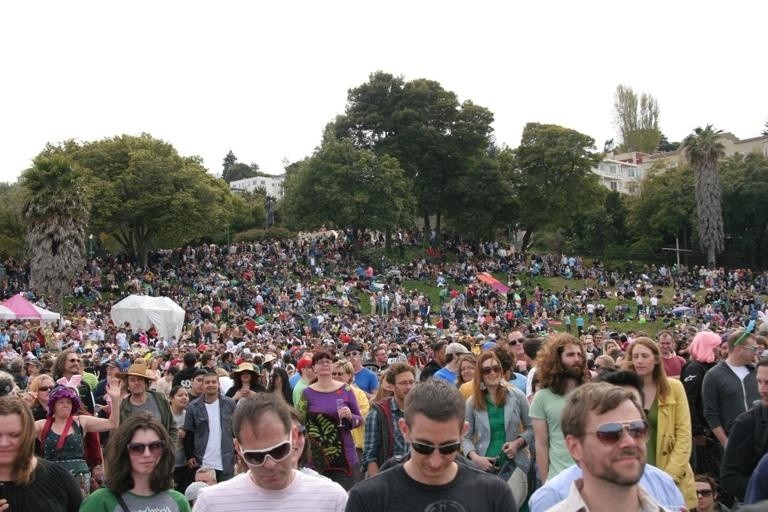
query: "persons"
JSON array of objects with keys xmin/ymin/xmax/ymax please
[{"xmin": 0, "ymin": 223, "xmax": 768, "ymax": 511}]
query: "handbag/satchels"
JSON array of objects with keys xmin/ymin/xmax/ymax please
[{"xmin": 488, "ymin": 451, "xmax": 518, "ymax": 482}]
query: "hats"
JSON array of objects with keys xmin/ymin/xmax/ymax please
[
  {"xmin": 296, "ymin": 342, "xmax": 474, "ymax": 371},
  {"xmin": 104, "ymin": 354, "xmax": 278, "ymax": 381},
  {"xmin": 47, "ymin": 385, "xmax": 80, "ymax": 415}
]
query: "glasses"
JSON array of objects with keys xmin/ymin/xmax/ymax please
[
  {"xmin": 695, "ymin": 489, "xmax": 714, "ymax": 498},
  {"xmin": 236, "ymin": 422, "xmax": 293, "ymax": 467},
  {"xmin": 332, "ymin": 372, "xmax": 343, "ymax": 376},
  {"xmin": 126, "ymin": 441, "xmax": 162, "ymax": 457},
  {"xmin": 742, "ymin": 344, "xmax": 759, "ymax": 353},
  {"xmin": 406, "ymin": 423, "xmax": 464, "ymax": 455},
  {"xmin": 507, "ymin": 337, "xmax": 525, "ymax": 346},
  {"xmin": 481, "ymin": 363, "xmax": 502, "ymax": 374},
  {"xmin": 69, "ymin": 358, "xmax": 80, "ymax": 363},
  {"xmin": 293, "ymin": 424, "xmax": 308, "ymax": 439},
  {"xmin": 582, "ymin": 420, "xmax": 652, "ymax": 447},
  {"xmin": 39, "ymin": 385, "xmax": 53, "ymax": 391}
]
